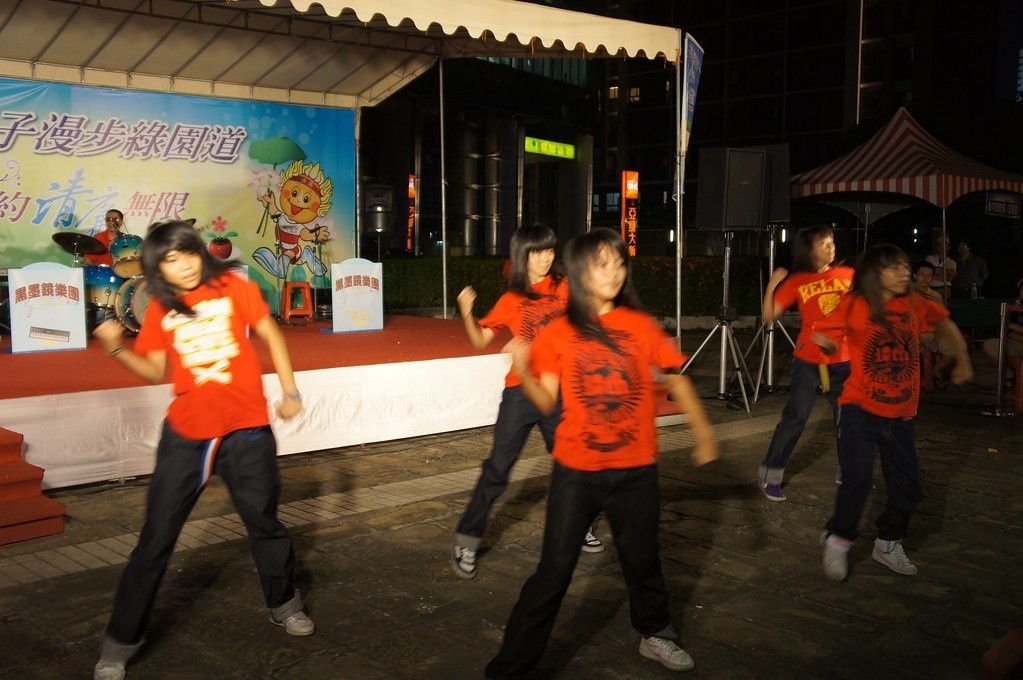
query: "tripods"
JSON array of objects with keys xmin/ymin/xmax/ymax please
[{"xmin": 677, "ymin": 226, "xmax": 795, "ymax": 418}]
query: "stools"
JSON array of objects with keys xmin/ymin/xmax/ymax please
[{"xmin": 283, "ymin": 282, "xmax": 313, "ymax": 324}]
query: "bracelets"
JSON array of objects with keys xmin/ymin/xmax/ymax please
[
  {"xmin": 286, "ymin": 389, "xmax": 301, "ymax": 399},
  {"xmin": 106, "ymin": 346, "xmax": 126, "ymax": 356}
]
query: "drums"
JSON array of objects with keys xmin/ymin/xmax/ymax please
[
  {"xmin": 113, "ymin": 276, "xmax": 155, "ymax": 332},
  {"xmin": 109, "ymin": 234, "xmax": 145, "ymax": 278},
  {"xmin": 81, "ymin": 264, "xmax": 123, "ymax": 309}
]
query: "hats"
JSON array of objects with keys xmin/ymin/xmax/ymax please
[{"xmin": 937, "ymin": 235, "xmax": 949, "ymax": 247}]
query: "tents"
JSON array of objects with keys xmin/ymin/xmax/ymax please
[
  {"xmin": 762, "ymin": 107, "xmax": 1023, "ymax": 316},
  {"xmin": 0, "ymin": 0, "xmax": 681, "ymax": 349}
]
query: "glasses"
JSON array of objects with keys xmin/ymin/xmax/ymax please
[
  {"xmin": 105, "ymin": 217, "xmax": 120, "ymax": 222},
  {"xmin": 917, "ymin": 272, "xmax": 933, "ymax": 280}
]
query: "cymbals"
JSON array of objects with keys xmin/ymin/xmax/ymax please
[{"xmin": 52, "ymin": 231, "xmax": 108, "ymax": 255}]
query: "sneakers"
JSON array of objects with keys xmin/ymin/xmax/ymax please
[
  {"xmin": 94, "ymin": 657, "xmax": 125, "ymax": 680},
  {"xmin": 452, "ymin": 539, "xmax": 476, "ymax": 579},
  {"xmin": 640, "ymin": 635, "xmax": 694, "ymax": 672},
  {"xmin": 758, "ymin": 477, "xmax": 786, "ymax": 502},
  {"xmin": 581, "ymin": 530, "xmax": 604, "ymax": 553},
  {"xmin": 819, "ymin": 531, "xmax": 850, "ymax": 581},
  {"xmin": 872, "ymin": 537, "xmax": 917, "ymax": 576},
  {"xmin": 268, "ymin": 609, "xmax": 315, "ymax": 636}
]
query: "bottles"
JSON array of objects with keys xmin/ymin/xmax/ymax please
[{"xmin": 971, "ymin": 282, "xmax": 977, "ymax": 300}]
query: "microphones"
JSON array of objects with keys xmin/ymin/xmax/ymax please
[
  {"xmin": 270, "ymin": 214, "xmax": 281, "ymax": 219},
  {"xmin": 114, "ymin": 218, "xmax": 119, "ymax": 227},
  {"xmin": 310, "ymin": 226, "xmax": 323, "ymax": 233}
]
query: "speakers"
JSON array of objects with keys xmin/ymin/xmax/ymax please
[
  {"xmin": 694, "ymin": 145, "xmax": 790, "ymax": 230},
  {"xmin": 363, "ymin": 185, "xmax": 396, "ymax": 236}
]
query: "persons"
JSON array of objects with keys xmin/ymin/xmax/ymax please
[
  {"xmin": 756, "ymin": 225, "xmax": 856, "ymax": 501},
  {"xmin": 84, "ymin": 210, "xmax": 125, "ymax": 267},
  {"xmin": 809, "ymin": 237, "xmax": 973, "ymax": 580},
  {"xmin": 452, "ymin": 227, "xmax": 604, "ymax": 578},
  {"xmin": 485, "ymin": 229, "xmax": 718, "ymax": 680},
  {"xmin": 93, "ymin": 221, "xmax": 315, "ymax": 680}
]
